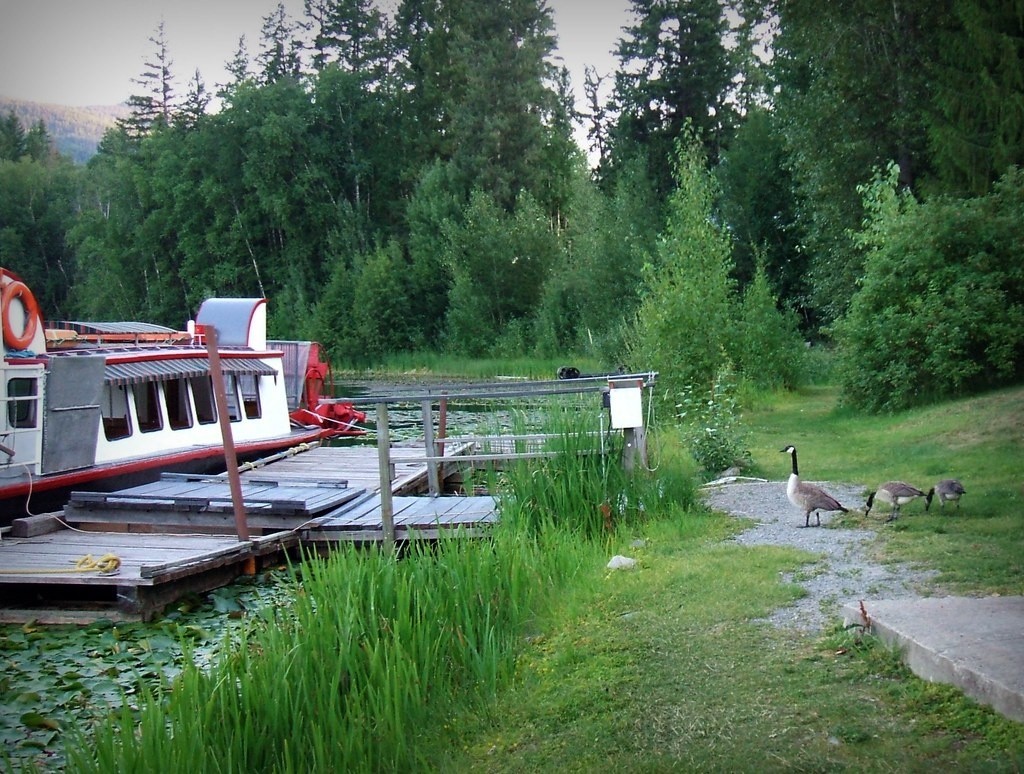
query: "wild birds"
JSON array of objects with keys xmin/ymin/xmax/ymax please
[
  {"xmin": 779, "ymin": 445, "xmax": 849, "ymax": 528},
  {"xmin": 865, "ymin": 480, "xmax": 967, "ymax": 526}
]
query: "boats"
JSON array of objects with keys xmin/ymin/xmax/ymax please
[{"xmin": 0, "ymin": 268, "xmax": 368, "ymax": 519}]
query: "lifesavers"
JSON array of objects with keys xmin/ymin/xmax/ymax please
[{"xmin": 2, "ymin": 281, "xmax": 38, "ymax": 349}]
